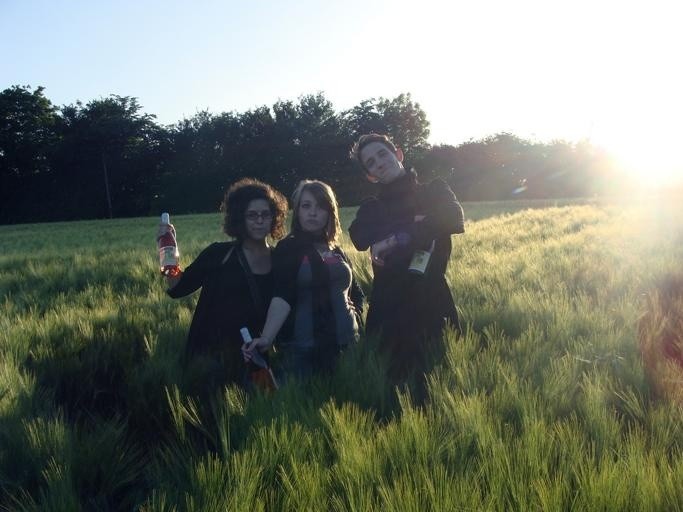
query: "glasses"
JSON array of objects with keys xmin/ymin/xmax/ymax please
[{"xmin": 242, "ymin": 211, "xmax": 276, "ymax": 220}]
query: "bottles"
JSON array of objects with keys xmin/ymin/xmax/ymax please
[
  {"xmin": 158, "ymin": 213, "xmax": 179, "ymax": 277},
  {"xmin": 239, "ymin": 327, "xmax": 280, "ymax": 394},
  {"xmin": 409, "ymin": 236, "xmax": 437, "ymax": 278}
]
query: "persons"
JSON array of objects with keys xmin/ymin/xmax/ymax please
[
  {"xmin": 239, "ymin": 177, "xmax": 365, "ymax": 375},
  {"xmin": 345, "ymin": 130, "xmax": 468, "ymax": 427},
  {"xmin": 155, "ymin": 177, "xmax": 289, "ymax": 388}
]
